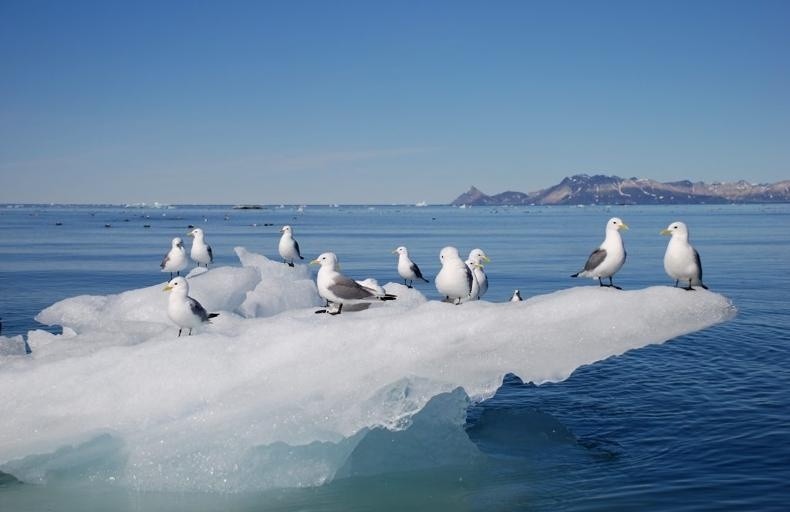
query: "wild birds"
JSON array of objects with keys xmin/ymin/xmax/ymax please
[
  {"xmin": 187, "ymin": 228, "xmax": 214, "ymax": 267},
  {"xmin": 310, "ymin": 252, "xmax": 397, "ymax": 315},
  {"xmin": 659, "ymin": 222, "xmax": 708, "ymax": 290},
  {"xmin": 279, "ymin": 225, "xmax": 304, "ymax": 267},
  {"xmin": 509, "ymin": 289, "xmax": 523, "ymax": 302},
  {"xmin": 162, "ymin": 276, "xmax": 220, "ymax": 338},
  {"xmin": 160, "ymin": 237, "xmax": 190, "ymax": 283},
  {"xmin": 434, "ymin": 246, "xmax": 491, "ymax": 305},
  {"xmin": 392, "ymin": 246, "xmax": 429, "ymax": 288},
  {"xmin": 570, "ymin": 217, "xmax": 629, "ymax": 290}
]
